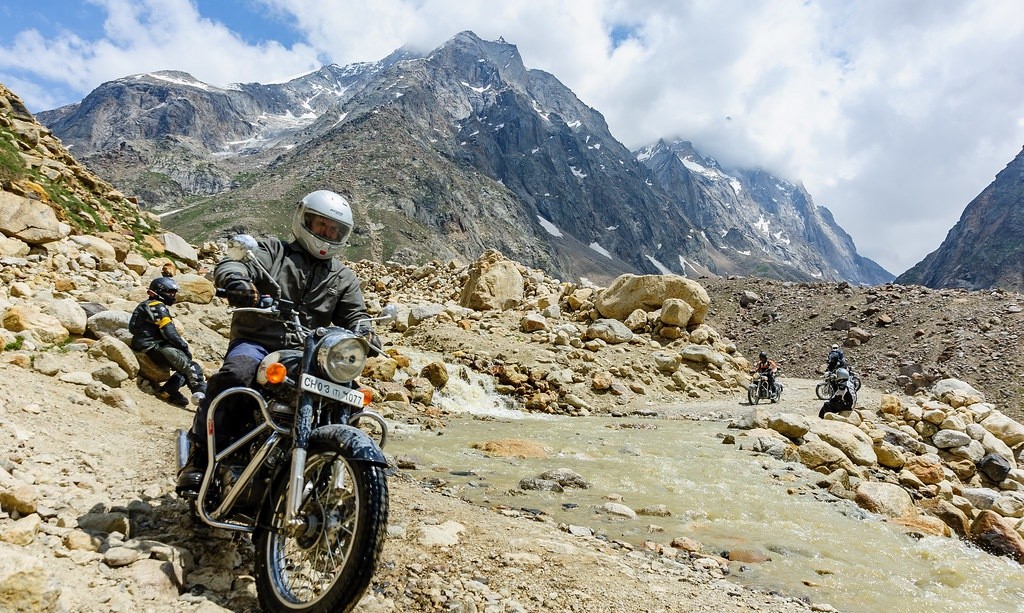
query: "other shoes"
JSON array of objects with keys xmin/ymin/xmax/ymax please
[
  {"xmin": 159, "ymin": 386, "xmax": 189, "ymax": 406},
  {"xmin": 772, "ymin": 392, "xmax": 776, "ymax": 397}
]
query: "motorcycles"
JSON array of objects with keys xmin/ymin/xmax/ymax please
[
  {"xmin": 174, "ymin": 234, "xmax": 390, "ymax": 613},
  {"xmin": 748, "ymin": 371, "xmax": 783, "ymax": 405},
  {"xmin": 815, "ymin": 365, "xmax": 861, "ymax": 400}
]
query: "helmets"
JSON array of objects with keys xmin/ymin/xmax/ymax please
[
  {"xmin": 759, "ymin": 352, "xmax": 768, "ymax": 361},
  {"xmin": 835, "ymin": 369, "xmax": 849, "ymax": 382},
  {"xmin": 292, "ymin": 190, "xmax": 354, "ymax": 260},
  {"xmin": 832, "ymin": 344, "xmax": 840, "ymax": 351},
  {"xmin": 148, "ymin": 278, "xmax": 177, "ymax": 306}
]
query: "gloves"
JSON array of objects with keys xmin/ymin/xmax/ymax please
[
  {"xmin": 227, "ymin": 279, "xmax": 258, "ymax": 308},
  {"xmin": 356, "ymin": 327, "xmax": 381, "ymax": 358}
]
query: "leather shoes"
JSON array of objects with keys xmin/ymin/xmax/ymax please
[{"xmin": 179, "ymin": 437, "xmax": 207, "ymax": 486}]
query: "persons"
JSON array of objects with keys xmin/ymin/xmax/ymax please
[
  {"xmin": 828, "ymin": 344, "xmax": 844, "ymax": 361},
  {"xmin": 825, "ymin": 352, "xmax": 847, "ymax": 375},
  {"xmin": 176, "ymin": 189, "xmax": 381, "ymax": 490},
  {"xmin": 128, "ymin": 277, "xmax": 208, "ymax": 405},
  {"xmin": 751, "ymin": 351, "xmax": 777, "ymax": 397},
  {"xmin": 818, "ymin": 368, "xmax": 856, "ymax": 419}
]
